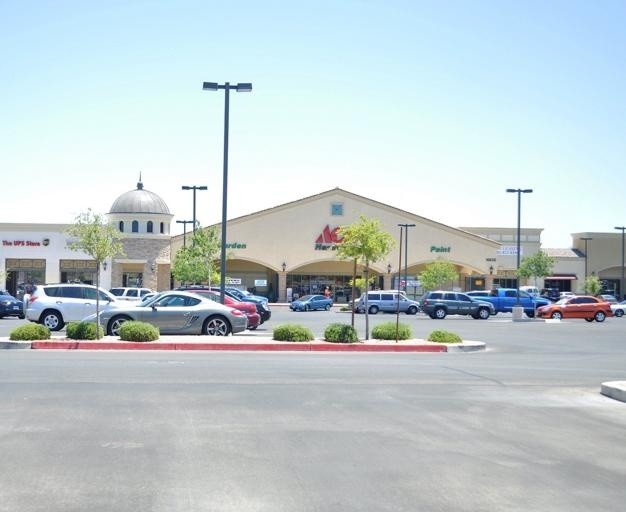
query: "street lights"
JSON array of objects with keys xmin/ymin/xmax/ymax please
[
  {"xmin": 200, "ymin": 76, "xmax": 253, "ymax": 307},
  {"xmin": 505, "ymin": 186, "xmax": 534, "ymax": 287},
  {"xmin": 613, "ymin": 225, "xmax": 626, "ymax": 280},
  {"xmin": 578, "ymin": 235, "xmax": 595, "ymax": 294},
  {"xmin": 398, "ymin": 221, "xmax": 419, "ymax": 293},
  {"xmin": 172, "ymin": 184, "xmax": 208, "ymax": 284}
]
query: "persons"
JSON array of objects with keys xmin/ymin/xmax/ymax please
[{"xmin": 324, "ymin": 287, "xmax": 332, "ymax": 298}]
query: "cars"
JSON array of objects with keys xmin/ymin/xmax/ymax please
[{"xmin": 0, "ymin": 292, "xmax": 23, "ymax": 320}]
